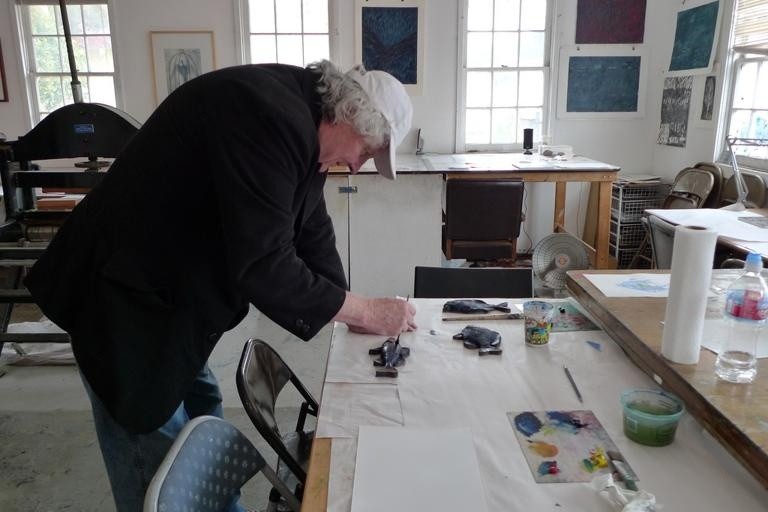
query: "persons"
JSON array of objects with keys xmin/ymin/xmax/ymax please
[{"xmin": 27, "ymin": 58, "xmax": 416, "ymax": 510}]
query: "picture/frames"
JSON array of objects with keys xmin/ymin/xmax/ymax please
[
  {"xmin": 150, "ymin": 29, "xmax": 217, "ymax": 108},
  {"xmin": 0, "ymin": 40, "xmax": 9, "ymax": 102},
  {"xmin": 663, "ymin": 0, "xmax": 723, "ymax": 77},
  {"xmin": 354, "ymin": 0, "xmax": 427, "ymax": 96},
  {"xmin": 556, "ymin": 47, "xmax": 647, "ymax": 118}
]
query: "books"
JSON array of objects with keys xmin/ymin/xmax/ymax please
[
  {"xmin": 39, "ymin": 198, "xmax": 81, "ymax": 207},
  {"xmin": 512, "ymin": 163, "xmax": 552, "ymax": 168}
]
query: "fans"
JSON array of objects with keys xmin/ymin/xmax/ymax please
[{"xmin": 532, "ymin": 233, "xmax": 590, "ymax": 300}]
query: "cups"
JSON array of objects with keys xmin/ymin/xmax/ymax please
[{"xmin": 522, "ymin": 302, "xmax": 553, "ymax": 346}]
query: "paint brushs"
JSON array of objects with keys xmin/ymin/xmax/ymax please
[
  {"xmin": 394, "ymin": 294, "xmax": 410, "ymax": 351},
  {"xmin": 607, "ymin": 450, "xmax": 653, "ymax": 512}
]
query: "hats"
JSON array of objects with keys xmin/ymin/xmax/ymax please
[{"xmin": 347, "ymin": 68, "xmax": 413, "ymax": 180}]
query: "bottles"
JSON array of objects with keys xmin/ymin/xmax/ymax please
[{"xmin": 713, "ymin": 253, "xmax": 768, "ymax": 383}]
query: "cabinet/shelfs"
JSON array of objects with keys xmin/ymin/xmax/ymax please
[{"xmin": 609, "ymin": 182, "xmax": 665, "ymax": 270}]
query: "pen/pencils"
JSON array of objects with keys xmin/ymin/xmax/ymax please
[{"xmin": 564, "ymin": 364, "xmax": 583, "ymax": 402}]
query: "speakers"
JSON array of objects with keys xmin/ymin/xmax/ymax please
[
  {"xmin": 523, "ymin": 129, "xmax": 532, "ymax": 154},
  {"xmin": 415, "ymin": 128, "xmax": 425, "ymax": 155}
]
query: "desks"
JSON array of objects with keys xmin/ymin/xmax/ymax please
[
  {"xmin": 561, "ymin": 264, "xmax": 768, "ymax": 486},
  {"xmin": 293, "ymin": 295, "xmax": 768, "ymax": 512},
  {"xmin": 326, "ymin": 147, "xmax": 620, "ymax": 271}
]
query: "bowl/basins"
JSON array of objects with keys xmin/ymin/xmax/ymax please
[{"xmin": 618, "ymin": 391, "xmax": 686, "ymax": 448}]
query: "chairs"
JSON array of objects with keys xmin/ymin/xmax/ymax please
[
  {"xmin": 624, "ymin": 162, "xmax": 766, "ymax": 270},
  {"xmin": 142, "ymin": 415, "xmax": 303, "ymax": 512},
  {"xmin": 442, "ymin": 176, "xmax": 525, "ymax": 266},
  {"xmin": 238, "ymin": 337, "xmax": 319, "ymax": 502},
  {"xmin": 412, "ymin": 265, "xmax": 537, "ymax": 298}
]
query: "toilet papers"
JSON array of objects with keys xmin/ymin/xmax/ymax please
[{"xmin": 661, "ymin": 225, "xmax": 721, "ymax": 366}]
